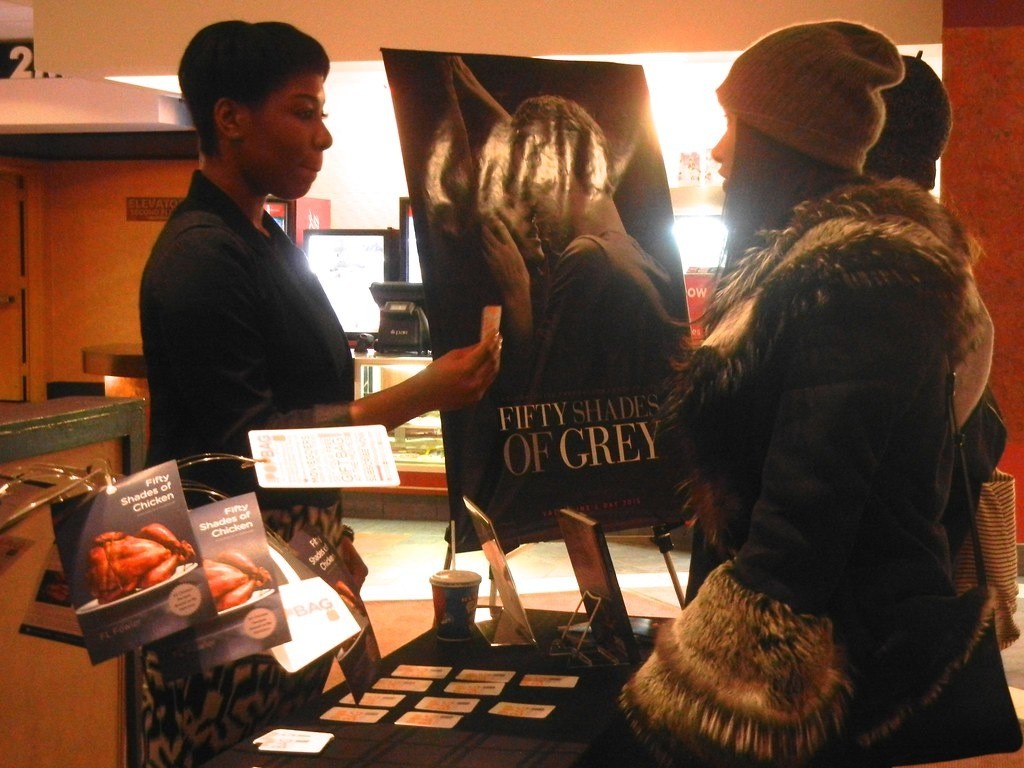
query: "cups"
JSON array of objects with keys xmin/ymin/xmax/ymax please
[
  {"xmin": 429, "ymin": 571, "xmax": 482, "ymax": 641},
  {"xmin": 681, "ymin": 272, "xmax": 715, "ymax": 340}
]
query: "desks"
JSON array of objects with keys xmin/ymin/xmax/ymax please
[{"xmin": 197, "ymin": 606, "xmax": 673, "ymax": 768}]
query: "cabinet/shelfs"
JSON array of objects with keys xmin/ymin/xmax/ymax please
[{"xmin": 353, "ymin": 353, "xmax": 446, "ymax": 474}]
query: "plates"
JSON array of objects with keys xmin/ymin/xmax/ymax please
[
  {"xmin": 217, "ymin": 588, "xmax": 276, "ymax": 616},
  {"xmin": 76, "ymin": 561, "xmax": 198, "ymax": 615}
]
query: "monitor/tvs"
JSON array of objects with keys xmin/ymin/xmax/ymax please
[{"xmin": 371, "ymin": 282, "xmax": 432, "ymax": 352}]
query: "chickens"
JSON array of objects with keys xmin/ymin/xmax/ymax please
[
  {"xmin": 202, "ymin": 549, "xmax": 273, "ymax": 612},
  {"xmin": 87, "ymin": 521, "xmax": 195, "ymax": 606}
]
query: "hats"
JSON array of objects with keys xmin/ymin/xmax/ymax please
[
  {"xmin": 718, "ymin": 20, "xmax": 906, "ymax": 175},
  {"xmin": 868, "ymin": 49, "xmax": 953, "ymax": 194}
]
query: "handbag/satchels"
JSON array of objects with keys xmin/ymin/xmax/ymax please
[
  {"xmin": 837, "ymin": 583, "xmax": 1023, "ymax": 768},
  {"xmin": 953, "ymin": 466, "xmax": 1020, "ymax": 651}
]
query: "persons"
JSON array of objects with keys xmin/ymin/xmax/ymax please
[
  {"xmin": 425, "ymin": 53, "xmax": 683, "ymax": 405},
  {"xmin": 138, "ymin": 21, "xmax": 503, "ymax": 768},
  {"xmin": 680, "ymin": 22, "xmax": 1024, "ymax": 768}
]
